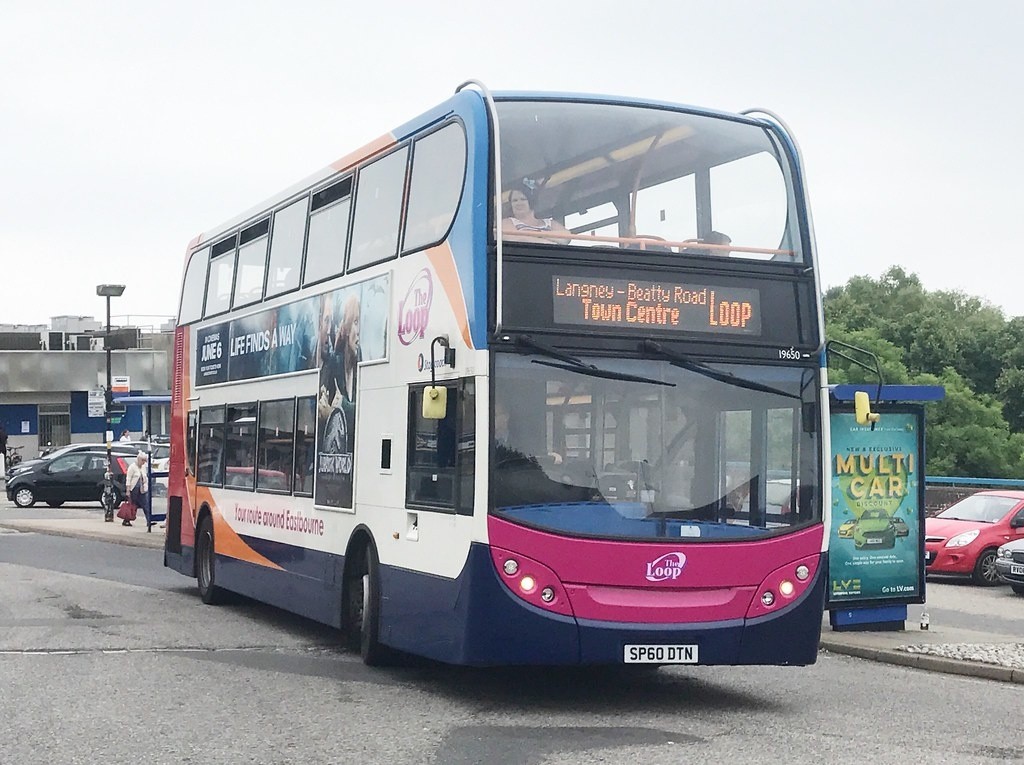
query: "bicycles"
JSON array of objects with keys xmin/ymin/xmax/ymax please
[{"xmin": 5, "ymin": 445, "xmax": 24, "ymax": 472}]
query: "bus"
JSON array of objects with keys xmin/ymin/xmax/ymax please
[{"xmin": 163, "ymin": 78, "xmax": 884, "ymax": 672}]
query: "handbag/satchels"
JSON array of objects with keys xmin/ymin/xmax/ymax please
[{"xmin": 117, "ymin": 497, "xmax": 137, "ymax": 521}]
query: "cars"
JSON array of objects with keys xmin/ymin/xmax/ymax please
[
  {"xmin": 5, "ymin": 451, "xmax": 139, "ymax": 510},
  {"xmin": 838, "ymin": 519, "xmax": 857, "ymax": 539},
  {"xmin": 924, "ymin": 490, "xmax": 1024, "ymax": 586},
  {"xmin": 891, "ymin": 517, "xmax": 909, "ymax": 536},
  {"xmin": 854, "ymin": 508, "xmax": 895, "ymax": 549},
  {"xmin": 995, "ymin": 538, "xmax": 1024, "ymax": 594}
]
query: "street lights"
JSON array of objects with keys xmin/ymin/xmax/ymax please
[{"xmin": 96, "ymin": 284, "xmax": 124, "ymax": 523}]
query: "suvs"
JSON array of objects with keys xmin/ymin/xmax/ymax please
[
  {"xmin": 4, "ymin": 443, "xmax": 139, "ymax": 483},
  {"xmin": 151, "ymin": 444, "xmax": 171, "ymax": 489}
]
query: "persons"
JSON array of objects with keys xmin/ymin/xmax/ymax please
[
  {"xmin": 689, "ymin": 230, "xmax": 732, "ymax": 258},
  {"xmin": 500, "ymin": 182, "xmax": 569, "ymax": 247},
  {"xmin": 120, "ymin": 430, "xmax": 131, "ymax": 442},
  {"xmin": 121, "ymin": 453, "xmax": 158, "ymax": 528},
  {"xmin": 493, "ymin": 400, "xmax": 562, "ymax": 473},
  {"xmin": 306, "ymin": 291, "xmax": 364, "ymax": 447}
]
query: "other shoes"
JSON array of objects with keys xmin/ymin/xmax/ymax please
[
  {"xmin": 122, "ymin": 519, "xmax": 132, "ymax": 527},
  {"xmin": 147, "ymin": 521, "xmax": 157, "ymax": 527}
]
